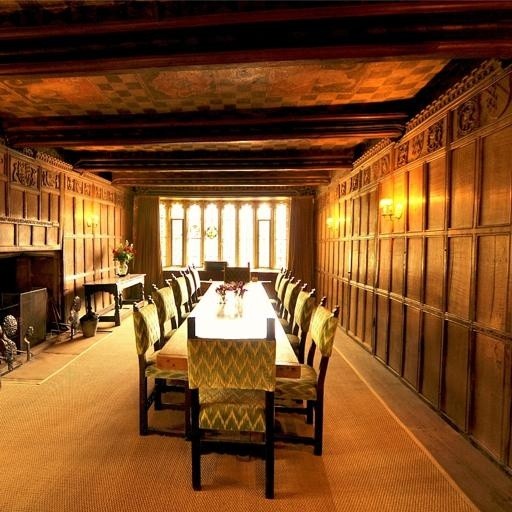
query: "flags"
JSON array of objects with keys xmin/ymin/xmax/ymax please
[
  {"xmin": 81, "ymin": 319, "xmax": 100, "ymax": 337},
  {"xmin": 118, "ymin": 263, "xmax": 127, "ymax": 275}
]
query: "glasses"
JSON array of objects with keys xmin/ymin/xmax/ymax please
[
  {"xmin": 92, "ymin": 213, "xmax": 101, "ymax": 230},
  {"xmin": 326, "ymin": 218, "xmax": 338, "ymax": 232},
  {"xmin": 380, "ymin": 199, "xmax": 399, "ymax": 219}
]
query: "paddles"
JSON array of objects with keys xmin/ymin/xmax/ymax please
[{"xmin": 0, "ymin": 281, "xmax": 480, "ymax": 512}]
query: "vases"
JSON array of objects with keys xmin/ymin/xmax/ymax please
[
  {"xmin": 112, "ymin": 240, "xmax": 135, "ymax": 270},
  {"xmin": 217, "ymin": 282, "xmax": 247, "ymax": 300}
]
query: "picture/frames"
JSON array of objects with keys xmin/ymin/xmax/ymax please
[{"xmin": 83, "ymin": 274, "xmax": 146, "ymax": 326}]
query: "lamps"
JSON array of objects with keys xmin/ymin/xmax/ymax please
[
  {"xmin": 151, "ymin": 279, "xmax": 186, "ymax": 393},
  {"xmin": 223, "ymin": 262, "xmax": 250, "ymax": 282},
  {"xmin": 273, "ymin": 270, "xmax": 292, "ymax": 317},
  {"xmin": 187, "ymin": 317, "xmax": 275, "ymax": 498},
  {"xmin": 187, "ymin": 263, "xmax": 203, "ymax": 300},
  {"xmin": 285, "ymin": 284, "xmax": 317, "ymax": 364},
  {"xmin": 133, "ymin": 296, "xmax": 188, "ymax": 436},
  {"xmin": 171, "ymin": 270, "xmax": 191, "ymax": 324},
  {"xmin": 274, "ymin": 295, "xmax": 339, "ymax": 456},
  {"xmin": 278, "ymin": 277, "xmax": 302, "ymax": 332},
  {"xmin": 269, "ymin": 267, "xmax": 286, "ymax": 305},
  {"xmin": 180, "ymin": 268, "xmax": 200, "ymax": 310}
]
